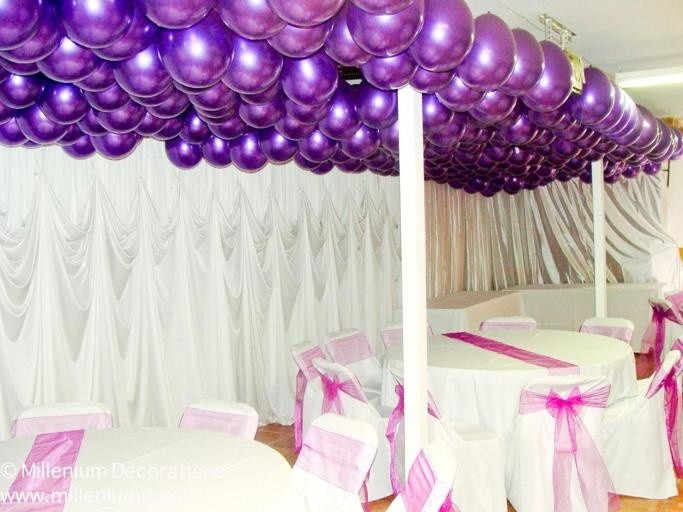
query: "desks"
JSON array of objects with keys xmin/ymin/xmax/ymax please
[
  {"xmin": 397, "ymin": 292, "xmax": 521, "ymax": 335},
  {"xmin": 0, "ymin": 427, "xmax": 308, "ymax": 512},
  {"xmin": 501, "ymin": 285, "xmax": 664, "ymax": 354}
]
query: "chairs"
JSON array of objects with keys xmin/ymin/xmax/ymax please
[{"xmin": 12, "ymin": 297, "xmax": 683, "ymax": 512}]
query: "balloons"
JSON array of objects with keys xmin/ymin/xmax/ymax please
[{"xmin": 2, "ymin": 0, "xmax": 683, "ymax": 199}]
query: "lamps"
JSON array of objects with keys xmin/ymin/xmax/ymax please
[{"xmin": 615, "ymin": 66, "xmax": 683, "ymax": 89}]
